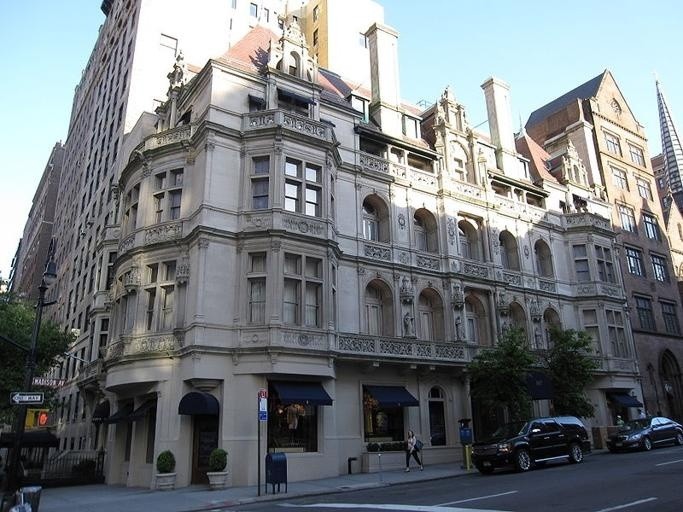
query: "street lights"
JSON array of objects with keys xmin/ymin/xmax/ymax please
[{"xmin": 2, "ymin": 234, "xmax": 58, "ymax": 507}]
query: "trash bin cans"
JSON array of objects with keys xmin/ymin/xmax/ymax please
[{"xmin": 591, "ymin": 425, "xmax": 607, "ymax": 449}]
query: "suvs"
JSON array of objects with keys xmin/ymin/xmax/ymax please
[{"xmin": 471, "ymin": 416, "xmax": 591, "ymax": 472}]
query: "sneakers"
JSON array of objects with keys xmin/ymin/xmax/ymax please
[
  {"xmin": 404, "ymin": 470, "xmax": 410, "ymax": 472},
  {"xmin": 421, "ymin": 465, "xmax": 424, "ymax": 471}
]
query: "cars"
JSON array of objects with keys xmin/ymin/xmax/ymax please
[{"xmin": 606, "ymin": 416, "xmax": 683, "ymax": 452}]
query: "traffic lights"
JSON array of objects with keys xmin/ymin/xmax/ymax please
[{"xmin": 26, "ymin": 409, "xmax": 48, "ymax": 428}]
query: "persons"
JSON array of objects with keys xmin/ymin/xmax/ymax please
[
  {"xmin": 616, "ymin": 415, "xmax": 625, "ymax": 428},
  {"xmin": 637, "ymin": 409, "xmax": 646, "ymax": 419},
  {"xmin": 404, "ymin": 431, "xmax": 423, "ymax": 472}
]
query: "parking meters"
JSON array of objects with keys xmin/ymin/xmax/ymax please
[{"xmin": 458, "ymin": 419, "xmax": 472, "ymax": 471}]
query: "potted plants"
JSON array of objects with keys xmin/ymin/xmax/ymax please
[
  {"xmin": 205, "ymin": 449, "xmax": 229, "ymax": 491},
  {"xmin": 155, "ymin": 450, "xmax": 177, "ymax": 491}
]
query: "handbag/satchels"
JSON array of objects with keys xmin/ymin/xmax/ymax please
[{"xmin": 414, "ymin": 440, "xmax": 423, "ymax": 450}]
